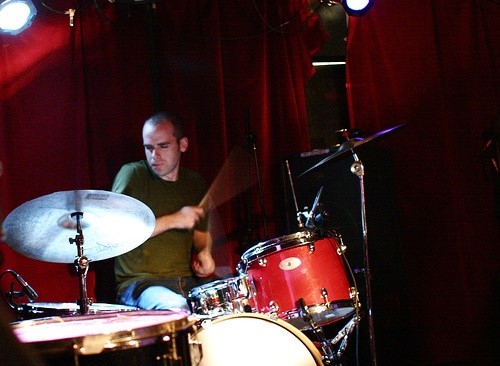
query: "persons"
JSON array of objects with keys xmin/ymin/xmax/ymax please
[{"xmin": 112, "ymin": 110, "xmax": 224, "ymax": 312}]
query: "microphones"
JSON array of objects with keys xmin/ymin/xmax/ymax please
[
  {"xmin": 282, "ymin": 147, "xmax": 338, "ymax": 161},
  {"xmin": 10, "ymin": 270, "xmax": 38, "ymax": 303},
  {"xmin": 305, "ymin": 186, "xmax": 324, "ymax": 229}
]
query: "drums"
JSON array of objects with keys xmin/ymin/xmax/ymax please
[
  {"xmin": 9, "ymin": 310, "xmax": 199, "ymax": 366},
  {"xmin": 237, "ymin": 227, "xmax": 363, "ymax": 330},
  {"xmin": 188, "ymin": 312, "xmax": 329, "ymax": 366},
  {"xmin": 188, "ymin": 276, "xmax": 250, "ymax": 312}
]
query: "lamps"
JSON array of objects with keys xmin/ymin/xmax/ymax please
[
  {"xmin": 341, "ymin": 0, "xmax": 375, "ymax": 16},
  {"xmin": 0, "ymin": 0, "xmax": 75, "ymax": 37}
]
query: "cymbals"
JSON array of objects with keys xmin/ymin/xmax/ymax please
[
  {"xmin": 300, "ymin": 143, "xmax": 342, "ymax": 157},
  {"xmin": 296, "ymin": 123, "xmax": 404, "ymax": 179},
  {"xmin": 302, "ymin": 187, "xmax": 325, "ymax": 226},
  {"xmin": 24, "ymin": 302, "xmax": 140, "ymax": 312},
  {"xmin": 1, "ymin": 189, "xmax": 157, "ymax": 264}
]
